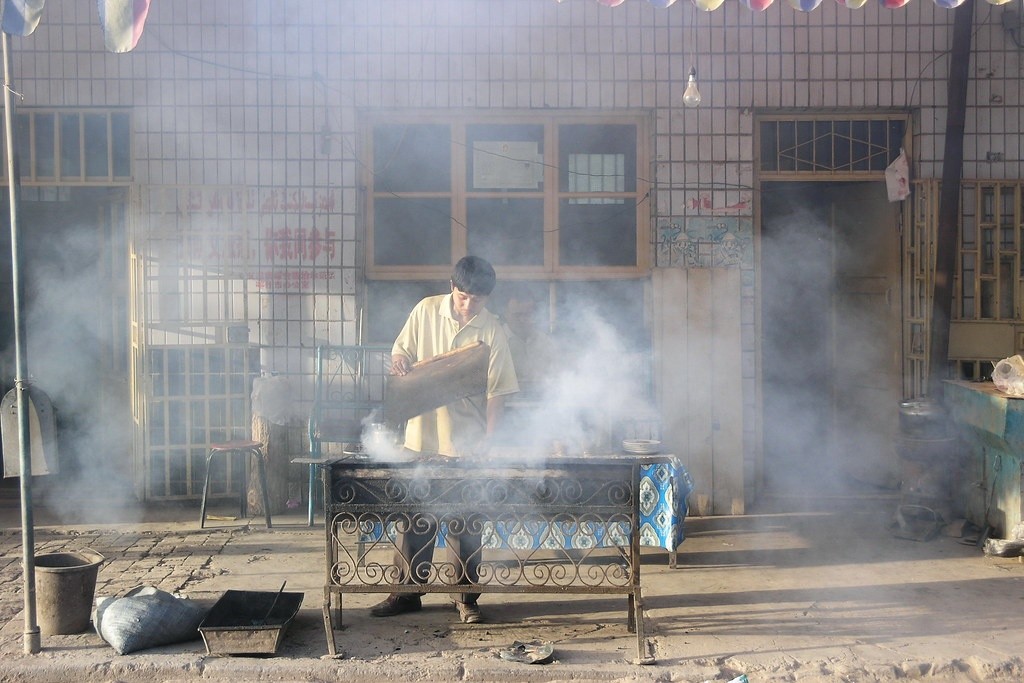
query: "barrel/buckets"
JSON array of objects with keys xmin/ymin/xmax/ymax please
[{"xmin": 20, "ymin": 546, "xmax": 106, "ymax": 635}]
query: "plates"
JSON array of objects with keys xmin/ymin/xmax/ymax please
[{"xmin": 622, "ymin": 439, "xmax": 663, "ymax": 455}]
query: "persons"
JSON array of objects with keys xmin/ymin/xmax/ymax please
[{"xmin": 367, "ymin": 256, "xmax": 521, "ymax": 623}]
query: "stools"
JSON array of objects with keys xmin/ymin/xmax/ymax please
[{"xmin": 198, "ymin": 440, "xmax": 273, "ymax": 532}]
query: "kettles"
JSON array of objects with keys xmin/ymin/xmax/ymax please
[{"xmin": 899, "ymin": 393, "xmax": 949, "ymax": 439}]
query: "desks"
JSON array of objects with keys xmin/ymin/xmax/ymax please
[{"xmin": 358, "ymin": 454, "xmax": 697, "ymax": 568}]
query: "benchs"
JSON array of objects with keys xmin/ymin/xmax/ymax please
[{"xmin": 306, "ymin": 344, "xmax": 391, "ymax": 527}]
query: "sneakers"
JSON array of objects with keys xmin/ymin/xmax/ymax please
[
  {"xmin": 370, "ymin": 591, "xmax": 421, "ymax": 617},
  {"xmin": 455, "ymin": 600, "xmax": 484, "ymax": 623}
]
